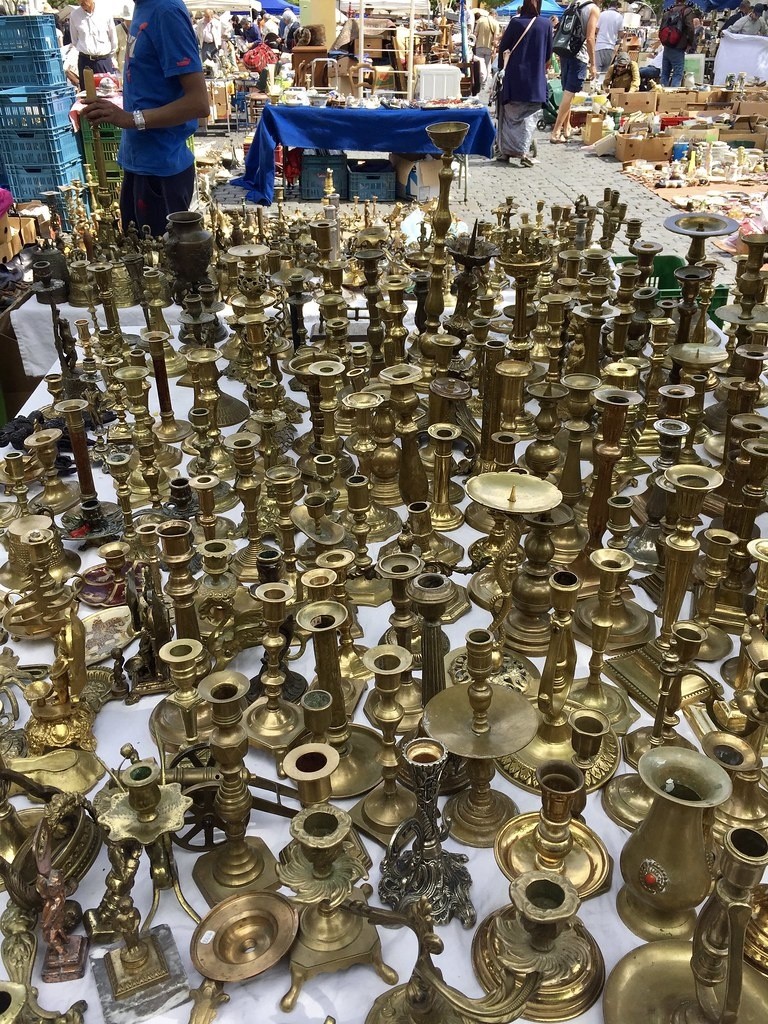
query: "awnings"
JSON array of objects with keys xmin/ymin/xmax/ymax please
[
  {"xmin": 230, "ymin": 11, "xmax": 251, "ymax": 15},
  {"xmin": 188, "ymin": 7, "xmax": 300, "ymax": 73}
]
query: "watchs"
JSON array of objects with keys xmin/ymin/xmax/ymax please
[{"xmin": 133, "ymin": 110, "xmax": 147, "ymax": 131}]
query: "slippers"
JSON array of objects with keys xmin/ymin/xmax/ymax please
[
  {"xmin": 550, "ymin": 135, "xmax": 569, "ymax": 143},
  {"xmin": 564, "ymin": 126, "xmax": 581, "ymax": 138}
]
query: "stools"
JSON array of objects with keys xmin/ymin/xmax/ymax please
[{"xmin": 250, "ymin": 92, "xmax": 268, "ymax": 124}]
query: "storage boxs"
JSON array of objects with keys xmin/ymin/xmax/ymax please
[
  {"xmin": 0, "ymin": 157, "xmax": 85, "ymax": 200},
  {"xmin": 0, "ymin": 212, "xmax": 12, "ymax": 244},
  {"xmin": 0, "ymin": 125, "xmax": 82, "ymax": 166},
  {"xmin": 388, "ymin": 152, "xmax": 443, "ymax": 201},
  {"xmin": 188, "ymin": 176, "xmax": 200, "ymax": 212},
  {"xmin": 611, "ymin": 254, "xmax": 729, "ymax": 331},
  {"xmin": 0, "ymin": 242, "xmax": 13, "ymax": 264},
  {"xmin": 346, "ymin": 159, "xmax": 396, "ymax": 202},
  {"xmin": 0, "ymin": 85, "xmax": 76, "ymax": 131},
  {"xmin": 11, "ymin": 235, "xmax": 23, "ymax": 256},
  {"xmin": 14, "ymin": 200, "xmax": 51, "ymax": 237},
  {"xmin": 92, "ymin": 181, "xmax": 122, "ymax": 209},
  {"xmin": 8, "ymin": 217, "xmax": 37, "ymax": 244},
  {"xmin": 299, "ymin": 148, "xmax": 347, "ymax": 200},
  {"xmin": 331, "ymin": 18, "xmax": 397, "ymax": 58},
  {"xmin": 0, "ymin": 48, "xmax": 67, "ymax": 89},
  {"xmin": 198, "ymin": 81, "xmax": 231, "ymax": 126},
  {"xmin": 79, "ymin": 114, "xmax": 122, "ymax": 143},
  {"xmin": 2, "ymin": 191, "xmax": 90, "ymax": 231},
  {"xmin": 570, "ymin": 83, "xmax": 768, "ymax": 162},
  {"xmin": 0, "ymin": 14, "xmax": 60, "ymax": 53},
  {"xmin": 83, "ymin": 135, "xmax": 196, "ymax": 181},
  {"xmin": 363, "ymin": 64, "xmax": 396, "ymax": 89},
  {"xmin": 414, "ymin": 64, "xmax": 461, "ymax": 100}
]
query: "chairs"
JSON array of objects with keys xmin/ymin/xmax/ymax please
[
  {"xmin": 349, "ymin": 63, "xmax": 377, "ymax": 98},
  {"xmin": 456, "ymin": 61, "xmax": 476, "ymax": 97}
]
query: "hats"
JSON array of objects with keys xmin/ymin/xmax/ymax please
[
  {"xmin": 120, "ymin": 3, "xmax": 134, "ymax": 20},
  {"xmin": 18, "ymin": 5, "xmax": 26, "ymax": 11},
  {"xmin": 617, "ymin": 52, "xmax": 630, "ymax": 65},
  {"xmin": 755, "ymin": 3, "xmax": 764, "ymax": 16}
]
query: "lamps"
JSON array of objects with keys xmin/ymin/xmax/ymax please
[{"xmin": 623, "ymin": 12, "xmax": 640, "ymax": 36}]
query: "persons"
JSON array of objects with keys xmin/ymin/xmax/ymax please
[
  {"xmin": 82, "ymin": 1, "xmax": 209, "ymax": 241},
  {"xmin": 494, "ymin": 0, "xmax": 552, "ymax": 166},
  {"xmin": 70, "ymin": 1, "xmax": 121, "ymax": 90},
  {"xmin": 19, "ymin": 6, "xmax": 27, "ymax": 15},
  {"xmin": 474, "ymin": 10, "xmax": 498, "ymax": 89},
  {"xmin": 40, "ymin": 4, "xmax": 63, "ymax": 48},
  {"xmin": 114, "ymin": 5, "xmax": 132, "ymax": 71},
  {"xmin": 554, "ymin": 0, "xmax": 768, "ymax": 93},
  {"xmin": 549, "ymin": 0, "xmax": 600, "ymax": 145}
]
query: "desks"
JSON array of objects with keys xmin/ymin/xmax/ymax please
[
  {"xmin": 414, "ymin": 31, "xmax": 443, "ymax": 61},
  {"xmin": 10, "ymin": 256, "xmax": 620, "ymax": 378},
  {"xmin": 0, "ymin": 320, "xmax": 768, "ymax": 1024},
  {"xmin": 229, "ymin": 98, "xmax": 497, "ymax": 206}
]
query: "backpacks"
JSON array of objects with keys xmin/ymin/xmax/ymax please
[
  {"xmin": 659, "ymin": 6, "xmax": 688, "ymax": 49},
  {"xmin": 553, "ymin": 0, "xmax": 594, "ymax": 57}
]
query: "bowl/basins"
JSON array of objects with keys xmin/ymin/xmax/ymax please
[
  {"xmin": 285, "ymin": 99, "xmax": 302, "ymax": 106},
  {"xmin": 307, "ymin": 95, "xmax": 328, "ymax": 108}
]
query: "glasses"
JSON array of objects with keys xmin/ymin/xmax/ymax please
[{"xmin": 618, "ymin": 65, "xmax": 626, "ymax": 68}]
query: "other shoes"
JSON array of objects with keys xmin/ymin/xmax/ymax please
[
  {"xmin": 522, "ymin": 158, "xmax": 535, "ymax": 167},
  {"xmin": 497, "ymin": 154, "xmax": 509, "ymax": 161}
]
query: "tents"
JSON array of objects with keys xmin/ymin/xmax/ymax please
[
  {"xmin": 497, "ymin": 0, "xmax": 565, "ymax": 18},
  {"xmin": 256, "ymin": 0, "xmax": 300, "ymax": 14}
]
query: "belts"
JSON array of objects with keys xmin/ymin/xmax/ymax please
[
  {"xmin": 80, "ymin": 52, "xmax": 110, "ymax": 61},
  {"xmin": 204, "ymin": 42, "xmax": 214, "ymax": 44}
]
